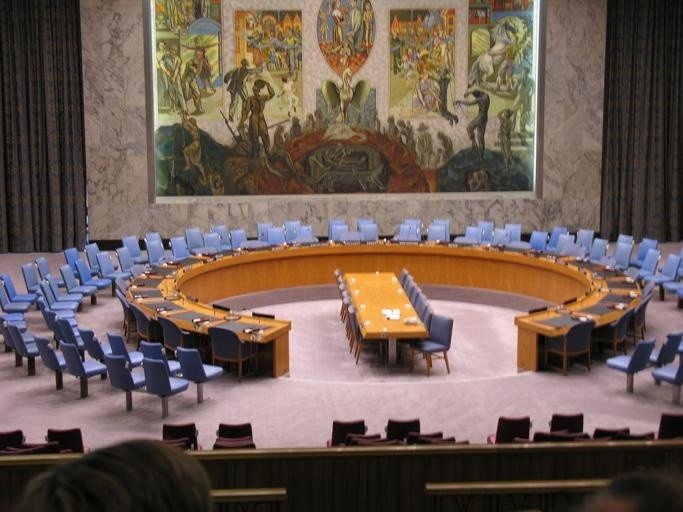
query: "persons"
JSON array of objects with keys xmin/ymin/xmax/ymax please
[
  {"xmin": 11, "ymin": 436, "xmax": 212, "ymax": 512},
  {"xmin": 568, "ymin": 473, "xmax": 683, "ymax": 512}
]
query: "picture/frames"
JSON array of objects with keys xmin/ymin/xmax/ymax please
[{"xmin": 143, "ymin": 2, "xmax": 546, "ymax": 202}]
